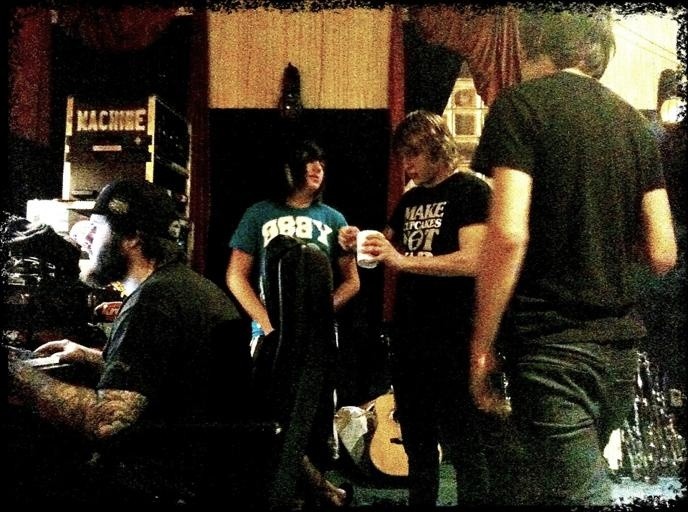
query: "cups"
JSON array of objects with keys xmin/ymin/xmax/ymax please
[{"xmin": 356, "ymin": 229, "xmax": 385, "ymax": 268}]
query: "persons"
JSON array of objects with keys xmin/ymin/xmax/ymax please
[
  {"xmin": 339, "ymin": 108, "xmax": 492, "ymax": 509},
  {"xmin": 0, "ymin": 178, "xmax": 249, "ymax": 511},
  {"xmin": 469, "ymin": 9, "xmax": 678, "ymax": 508},
  {"xmin": 223, "ymin": 142, "xmax": 362, "ymax": 460}
]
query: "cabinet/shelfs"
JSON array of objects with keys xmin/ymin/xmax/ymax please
[{"xmin": 442, "ymin": 77, "xmax": 487, "ymax": 146}]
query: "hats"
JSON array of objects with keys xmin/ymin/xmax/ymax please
[{"xmin": 67, "ymin": 174, "xmax": 177, "ymax": 224}]
